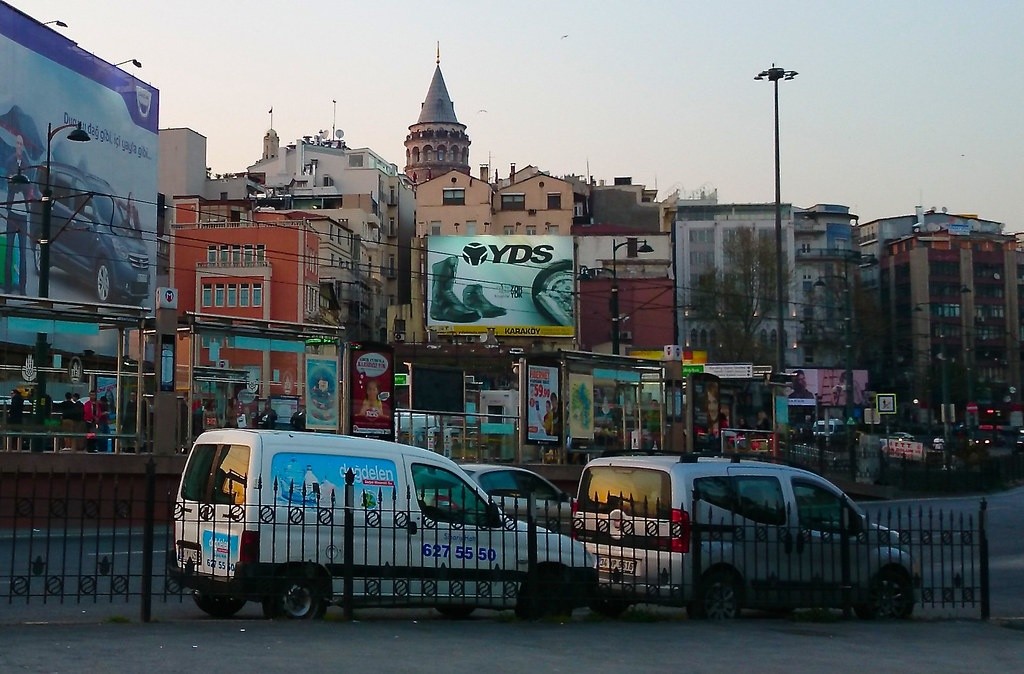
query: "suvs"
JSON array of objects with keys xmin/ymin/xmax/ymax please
[{"xmin": 571, "ymin": 448, "xmax": 919, "ymax": 620}]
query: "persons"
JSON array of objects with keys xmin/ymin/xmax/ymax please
[
  {"xmin": 648, "ymin": 400, "xmax": 661, "ymax": 451},
  {"xmin": 789, "ymin": 371, "xmax": 814, "ymax": 399},
  {"xmin": 8, "ymin": 389, "xmax": 48, "ymax": 450},
  {"xmin": 193, "ymin": 398, "xmax": 248, "ymax": 437},
  {"xmin": 543, "ymin": 393, "xmax": 559, "ymax": 436},
  {"xmin": 792, "ymin": 426, "xmax": 817, "ymax": 451},
  {"xmin": 361, "ymin": 380, "xmax": 383, "ymax": 416},
  {"xmin": 738, "ymin": 418, "xmax": 752, "ymax": 434},
  {"xmin": 832, "ymin": 372, "xmax": 863, "ymax": 404},
  {"xmin": 707, "ymin": 384, "xmax": 719, "ymax": 438},
  {"xmin": 849, "ymin": 432, "xmax": 858, "ymax": 481},
  {"xmin": 52, "ymin": 391, "xmax": 109, "ymax": 453},
  {"xmin": 6, "ymin": 135, "xmax": 31, "ymax": 296},
  {"xmin": 720, "ymin": 414, "xmax": 730, "ymax": 439},
  {"xmin": 115, "ymin": 192, "xmax": 141, "ymax": 238},
  {"xmin": 122, "ymin": 390, "xmax": 147, "ymax": 453},
  {"xmin": 261, "ymin": 403, "xmax": 278, "ymax": 430},
  {"xmin": 757, "ymin": 420, "xmax": 770, "ymax": 435},
  {"xmin": 290, "ymin": 406, "xmax": 306, "ymax": 430}
]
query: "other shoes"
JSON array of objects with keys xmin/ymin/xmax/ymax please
[{"xmin": 63, "ymin": 447, "xmax": 72, "ymax": 451}]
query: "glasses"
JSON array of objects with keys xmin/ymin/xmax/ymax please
[{"xmin": 10, "ymin": 392, "xmax": 15, "ymax": 394}]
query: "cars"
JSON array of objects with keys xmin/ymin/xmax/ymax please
[
  {"xmin": 889, "ymin": 432, "xmax": 915, "ymax": 442},
  {"xmin": 1015, "ymin": 427, "xmax": 1024, "ymax": 452},
  {"xmin": 569, "ymin": 417, "xmax": 616, "ymax": 447},
  {"xmin": 415, "ymin": 464, "xmax": 576, "ymax": 535},
  {"xmin": 29, "ymin": 161, "xmax": 150, "ymax": 305},
  {"xmin": 641, "ymin": 413, "xmax": 724, "ymax": 454},
  {"xmin": 392, "ymin": 412, "xmax": 439, "ymax": 442},
  {"xmin": 812, "ymin": 419, "xmax": 843, "ymax": 438}
]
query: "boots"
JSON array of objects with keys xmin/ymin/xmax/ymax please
[{"xmin": 430, "ymin": 256, "xmax": 481, "ymax": 323}]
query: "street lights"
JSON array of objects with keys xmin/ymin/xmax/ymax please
[
  {"xmin": 577, "ymin": 239, "xmax": 654, "ymax": 355},
  {"xmin": 812, "ymin": 253, "xmax": 879, "ymax": 482},
  {"xmin": 754, "ymin": 63, "xmax": 799, "ymax": 372},
  {"xmin": 9, "ymin": 123, "xmax": 91, "ymax": 451},
  {"xmin": 913, "ymin": 284, "xmax": 972, "ymax": 462}
]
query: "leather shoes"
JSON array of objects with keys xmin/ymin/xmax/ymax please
[{"xmin": 463, "ymin": 285, "xmax": 507, "ymax": 318}]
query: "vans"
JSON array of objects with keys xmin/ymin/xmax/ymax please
[{"xmin": 166, "ymin": 429, "xmax": 598, "ymax": 621}]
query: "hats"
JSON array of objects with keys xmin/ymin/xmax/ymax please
[{"xmin": 130, "ymin": 392, "xmax": 135, "ymax": 395}]
